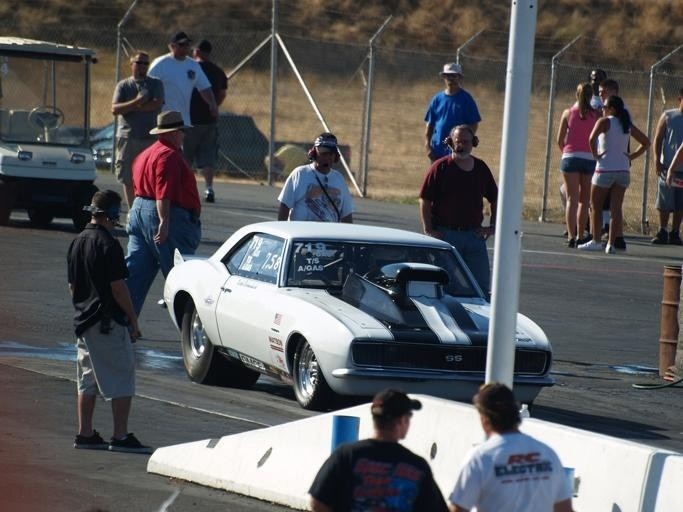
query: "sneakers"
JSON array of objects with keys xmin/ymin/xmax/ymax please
[
  {"xmin": 563, "ymin": 239, "xmax": 574, "ymax": 249},
  {"xmin": 577, "ymin": 240, "xmax": 601, "ymax": 251},
  {"xmin": 575, "ymin": 235, "xmax": 586, "ymax": 245},
  {"xmin": 204, "ymin": 190, "xmax": 213, "ymax": 201},
  {"xmin": 668, "ymin": 231, "xmax": 680, "ymax": 244},
  {"xmin": 605, "ymin": 244, "xmax": 614, "ymax": 254},
  {"xmin": 108, "ymin": 433, "xmax": 152, "ymax": 454},
  {"xmin": 649, "ymin": 228, "xmax": 667, "ymax": 243},
  {"xmin": 73, "ymin": 429, "xmax": 109, "ymax": 450},
  {"xmin": 614, "ymin": 237, "xmax": 625, "ymax": 249}
]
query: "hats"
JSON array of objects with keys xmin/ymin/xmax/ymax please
[
  {"xmin": 148, "ymin": 110, "xmax": 195, "ymax": 135},
  {"xmin": 473, "ymin": 384, "xmax": 517, "ymax": 418},
  {"xmin": 371, "ymin": 388, "xmax": 423, "ymax": 418},
  {"xmin": 171, "ymin": 31, "xmax": 190, "ymax": 43},
  {"xmin": 315, "ymin": 133, "xmax": 336, "ymax": 155},
  {"xmin": 438, "ymin": 64, "xmax": 463, "ymax": 78}
]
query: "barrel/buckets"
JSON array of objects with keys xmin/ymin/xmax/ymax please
[{"xmin": 659, "ymin": 263, "xmax": 683, "ymax": 381}]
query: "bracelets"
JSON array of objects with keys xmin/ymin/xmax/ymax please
[{"xmin": 490, "ymin": 223, "xmax": 494, "ymax": 228}]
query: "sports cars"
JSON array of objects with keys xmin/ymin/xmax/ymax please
[{"xmin": 163, "ymin": 222, "xmax": 555, "ymax": 411}]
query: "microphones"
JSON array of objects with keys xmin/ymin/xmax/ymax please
[
  {"xmin": 110, "ymin": 218, "xmax": 125, "ymax": 229},
  {"xmin": 448, "ymin": 144, "xmax": 461, "ymax": 155},
  {"xmin": 310, "ymin": 157, "xmax": 328, "ymax": 170}
]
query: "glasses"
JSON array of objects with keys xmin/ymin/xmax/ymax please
[
  {"xmin": 133, "ymin": 61, "xmax": 149, "ymax": 65},
  {"xmin": 190, "ymin": 39, "xmax": 211, "ymax": 52},
  {"xmin": 176, "ymin": 44, "xmax": 188, "ymax": 47},
  {"xmin": 600, "ymin": 104, "xmax": 606, "ymax": 110}
]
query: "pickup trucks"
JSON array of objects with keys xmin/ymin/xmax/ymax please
[{"xmin": 89, "ymin": 114, "xmax": 351, "ymax": 185}]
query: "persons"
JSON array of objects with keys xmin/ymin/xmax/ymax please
[
  {"xmin": 447, "ymin": 382, "xmax": 572, "ymax": 512},
  {"xmin": 555, "ymin": 68, "xmax": 683, "ymax": 254},
  {"xmin": 109, "ymin": 51, "xmax": 165, "ymax": 211},
  {"xmin": 307, "ymin": 386, "xmax": 450, "ymax": 512},
  {"xmin": 124, "ymin": 109, "xmax": 202, "ymax": 335},
  {"xmin": 424, "ymin": 63, "xmax": 482, "ymax": 165},
  {"xmin": 190, "ymin": 38, "xmax": 228, "ymax": 203},
  {"xmin": 66, "ymin": 187, "xmax": 152, "ymax": 455},
  {"xmin": 276, "ymin": 132, "xmax": 356, "ymax": 223},
  {"xmin": 417, "ymin": 126, "xmax": 498, "ymax": 302},
  {"xmin": 146, "ymin": 34, "xmax": 220, "ymax": 170}
]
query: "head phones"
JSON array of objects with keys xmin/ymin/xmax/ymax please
[
  {"xmin": 444, "ymin": 133, "xmax": 480, "ymax": 150},
  {"xmin": 80, "ymin": 204, "xmax": 122, "ymax": 223},
  {"xmin": 306, "ymin": 142, "xmax": 341, "ymax": 164}
]
